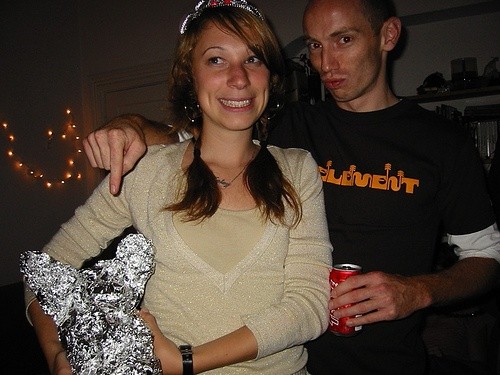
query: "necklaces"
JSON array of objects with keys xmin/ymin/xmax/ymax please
[{"xmin": 215, "ymin": 146, "xmax": 257, "ymax": 188}]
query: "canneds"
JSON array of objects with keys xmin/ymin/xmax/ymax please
[{"xmin": 328, "ymin": 263, "xmax": 364, "ymax": 336}]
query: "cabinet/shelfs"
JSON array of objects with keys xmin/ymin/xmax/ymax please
[{"xmin": 405, "ymin": 84, "xmax": 500, "ymax": 180}]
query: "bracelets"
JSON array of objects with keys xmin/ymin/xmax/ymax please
[{"xmin": 178, "ymin": 344, "xmax": 194, "ymax": 375}]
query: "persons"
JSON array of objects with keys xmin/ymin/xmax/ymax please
[
  {"xmin": 23, "ymin": 0, "xmax": 334, "ymax": 375},
  {"xmin": 82, "ymin": 1, "xmax": 500, "ymax": 375}
]
